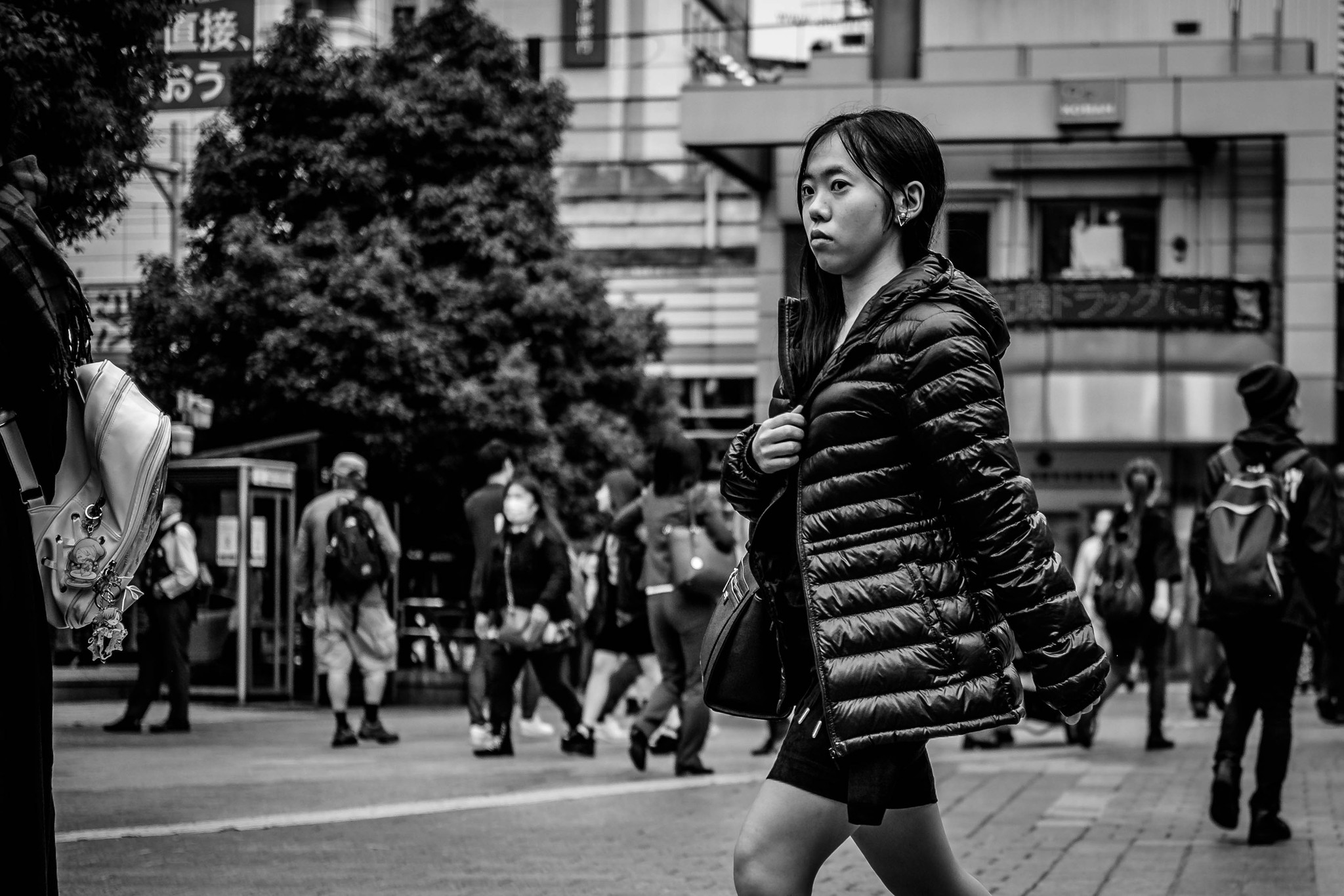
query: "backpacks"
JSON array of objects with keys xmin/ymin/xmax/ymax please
[
  {"xmin": 1202, "ymin": 444, "xmax": 1310, "ymax": 630},
  {"xmin": 1093, "ymin": 513, "xmax": 1144, "ymax": 628},
  {"xmin": 323, "ymin": 496, "xmax": 394, "ymax": 605},
  {"xmin": 0, "ymin": 361, "xmax": 172, "ymax": 663},
  {"xmin": 192, "ymin": 562, "xmax": 211, "ymax": 611}
]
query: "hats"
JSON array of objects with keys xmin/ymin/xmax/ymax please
[
  {"xmin": 332, "ymin": 454, "xmax": 367, "ymax": 482},
  {"xmin": 1236, "ymin": 365, "xmax": 1300, "ymax": 417}
]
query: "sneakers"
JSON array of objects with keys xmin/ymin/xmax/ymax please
[{"xmin": 468, "ymin": 715, "xmax": 629, "ymax": 757}]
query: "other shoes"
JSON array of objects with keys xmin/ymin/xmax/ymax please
[
  {"xmin": 676, "ymin": 763, "xmax": 715, "ymax": 778},
  {"xmin": 629, "ymin": 728, "xmax": 648, "ymax": 771},
  {"xmin": 1249, "ymin": 812, "xmax": 1289, "ymax": 845},
  {"xmin": 1210, "ymin": 760, "xmax": 1240, "ymax": 830},
  {"xmin": 1147, "ymin": 717, "xmax": 1175, "ymax": 751},
  {"xmin": 104, "ymin": 715, "xmax": 142, "ymax": 735},
  {"xmin": 150, "ymin": 714, "xmax": 190, "ymax": 733}
]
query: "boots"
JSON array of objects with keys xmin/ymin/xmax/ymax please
[
  {"xmin": 332, "ymin": 711, "xmax": 357, "ymax": 747},
  {"xmin": 359, "ymin": 704, "xmax": 400, "ymax": 744}
]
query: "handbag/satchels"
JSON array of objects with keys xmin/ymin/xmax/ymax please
[
  {"xmin": 669, "ymin": 526, "xmax": 736, "ymax": 597},
  {"xmin": 499, "ymin": 607, "xmax": 546, "ymax": 651}
]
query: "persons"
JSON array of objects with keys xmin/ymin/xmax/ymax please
[
  {"xmin": 101, "ymin": 488, "xmax": 197, "ymax": 736},
  {"xmin": 961, "ymin": 361, "xmax": 1344, "ymax": 847},
  {"xmin": 721, "ymin": 110, "xmax": 1109, "ymax": 896},
  {"xmin": 225, "ymin": 440, "xmax": 788, "ymax": 778},
  {"xmin": 0, "ymin": 153, "xmax": 93, "ymax": 896}
]
